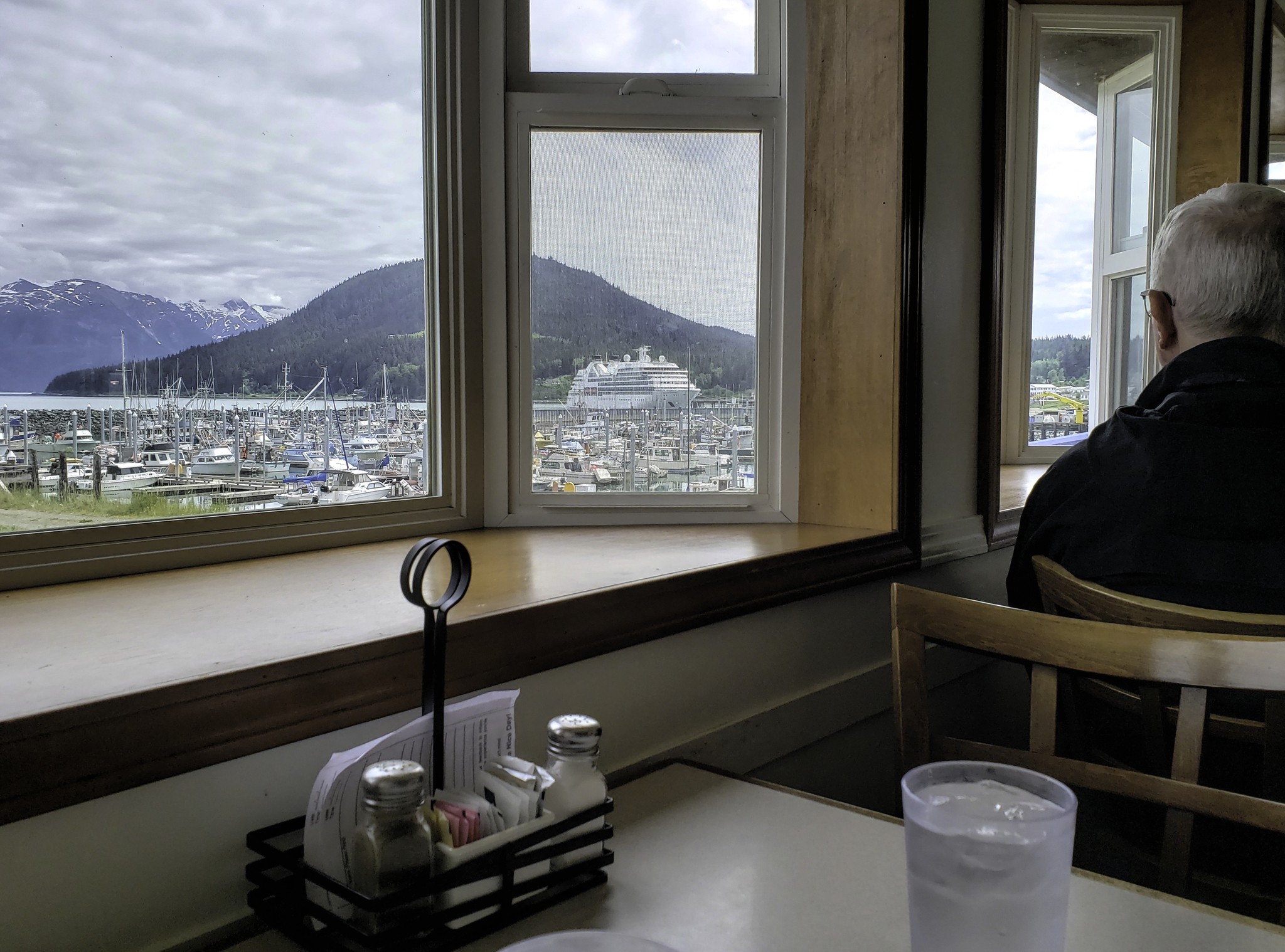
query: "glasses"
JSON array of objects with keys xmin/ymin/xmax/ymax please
[{"xmin": 1140, "ymin": 288, "xmax": 1173, "ymax": 318}]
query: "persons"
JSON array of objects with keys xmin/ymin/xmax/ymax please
[{"xmin": 1004, "ymin": 181, "xmax": 1285, "ymax": 930}]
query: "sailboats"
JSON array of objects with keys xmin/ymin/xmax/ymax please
[
  {"xmin": 0, "ymin": 328, "xmax": 427, "ymax": 509},
  {"xmin": 531, "ymin": 348, "xmax": 755, "ymax": 492}
]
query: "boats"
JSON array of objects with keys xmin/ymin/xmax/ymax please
[{"xmin": 566, "ymin": 343, "xmax": 701, "ymax": 408}]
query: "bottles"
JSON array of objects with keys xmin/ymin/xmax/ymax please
[
  {"xmin": 540, "ymin": 714, "xmax": 608, "ymax": 873},
  {"xmin": 349, "ymin": 757, "xmax": 436, "ymax": 939}
]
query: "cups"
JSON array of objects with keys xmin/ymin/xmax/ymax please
[
  {"xmin": 900, "ymin": 759, "xmax": 1079, "ymax": 952},
  {"xmin": 499, "ymin": 930, "xmax": 679, "ymax": 952}
]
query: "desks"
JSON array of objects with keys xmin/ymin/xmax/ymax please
[{"xmin": 226, "ymin": 758, "xmax": 1285, "ymax": 952}]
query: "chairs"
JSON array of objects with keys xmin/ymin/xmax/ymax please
[
  {"xmin": 1031, "ymin": 556, "xmax": 1285, "ymax": 776},
  {"xmin": 890, "ymin": 583, "xmax": 1285, "ymax": 914}
]
